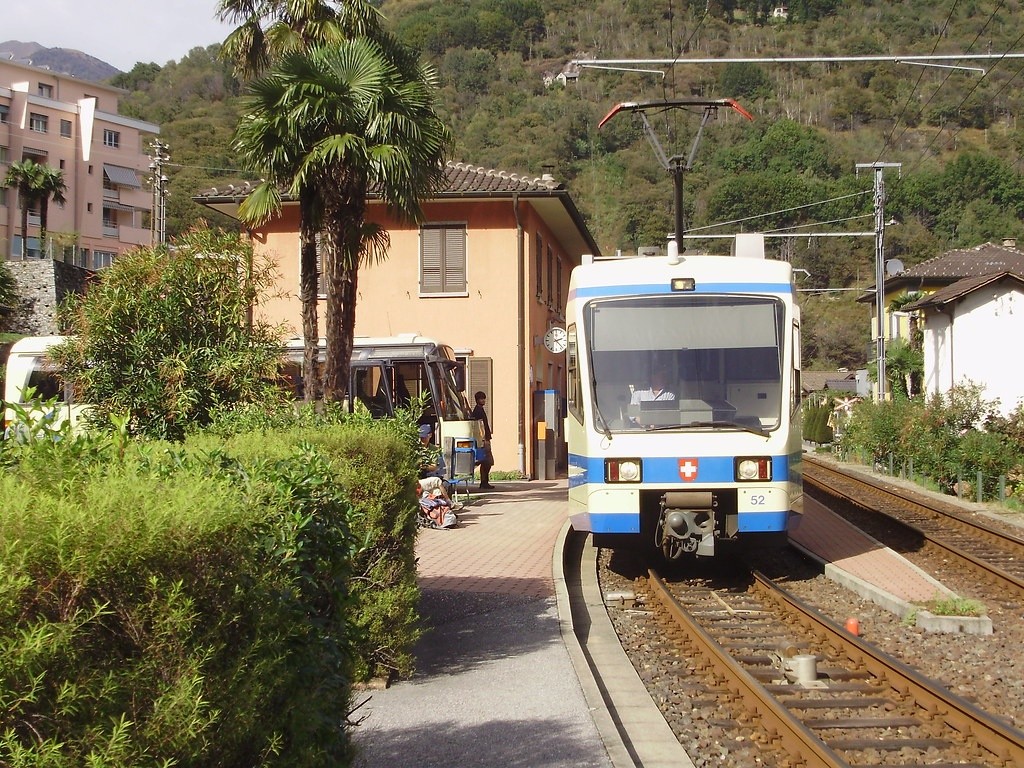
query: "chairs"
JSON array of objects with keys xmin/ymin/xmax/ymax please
[{"xmin": 439, "ymin": 447, "xmax": 476, "ymax": 508}]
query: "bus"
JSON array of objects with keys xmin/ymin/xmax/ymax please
[{"xmin": 5, "ymin": 335, "xmax": 490, "ymax": 492}]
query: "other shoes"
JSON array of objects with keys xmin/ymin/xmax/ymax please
[{"xmin": 479, "ymin": 483, "xmax": 494, "ymax": 489}]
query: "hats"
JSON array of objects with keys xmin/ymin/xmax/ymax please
[
  {"xmin": 475, "ymin": 392, "xmax": 486, "ymax": 400},
  {"xmin": 418, "ymin": 424, "xmax": 431, "ymax": 438}
]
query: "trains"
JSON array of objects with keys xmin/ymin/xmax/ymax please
[{"xmin": 562, "ymin": 232, "xmax": 806, "ymax": 569}]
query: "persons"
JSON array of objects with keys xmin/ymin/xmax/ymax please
[
  {"xmin": 38, "ymin": 380, "xmax": 52, "ymax": 402},
  {"xmin": 628, "ymin": 364, "xmax": 678, "ymax": 428},
  {"xmin": 415, "ymin": 423, "xmax": 463, "ymax": 530},
  {"xmin": 472, "ymin": 391, "xmax": 496, "ymax": 490},
  {"xmin": 355, "ymin": 371, "xmax": 374, "ymax": 418}
]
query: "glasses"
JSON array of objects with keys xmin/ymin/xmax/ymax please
[{"xmin": 480, "ymin": 397, "xmax": 485, "ymax": 399}]
